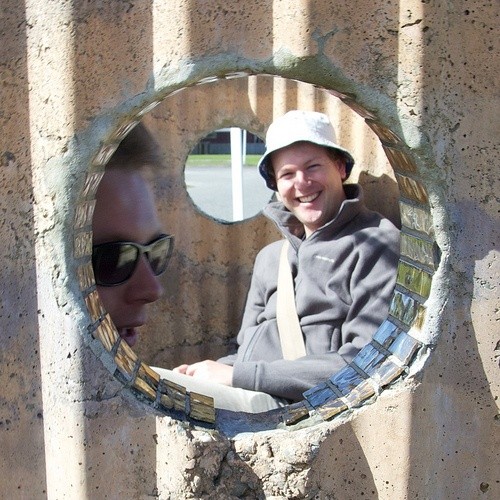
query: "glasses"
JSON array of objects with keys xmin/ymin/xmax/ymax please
[{"xmin": 92, "ymin": 235, "xmax": 175, "ymax": 287}]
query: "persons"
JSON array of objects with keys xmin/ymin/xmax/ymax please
[
  {"xmin": 172, "ymin": 109, "xmax": 402, "ymax": 409},
  {"xmin": 91, "ymin": 124, "xmax": 173, "ymax": 353}
]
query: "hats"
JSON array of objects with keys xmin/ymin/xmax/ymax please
[{"xmin": 258, "ymin": 110, "xmax": 354, "ymax": 192}]
